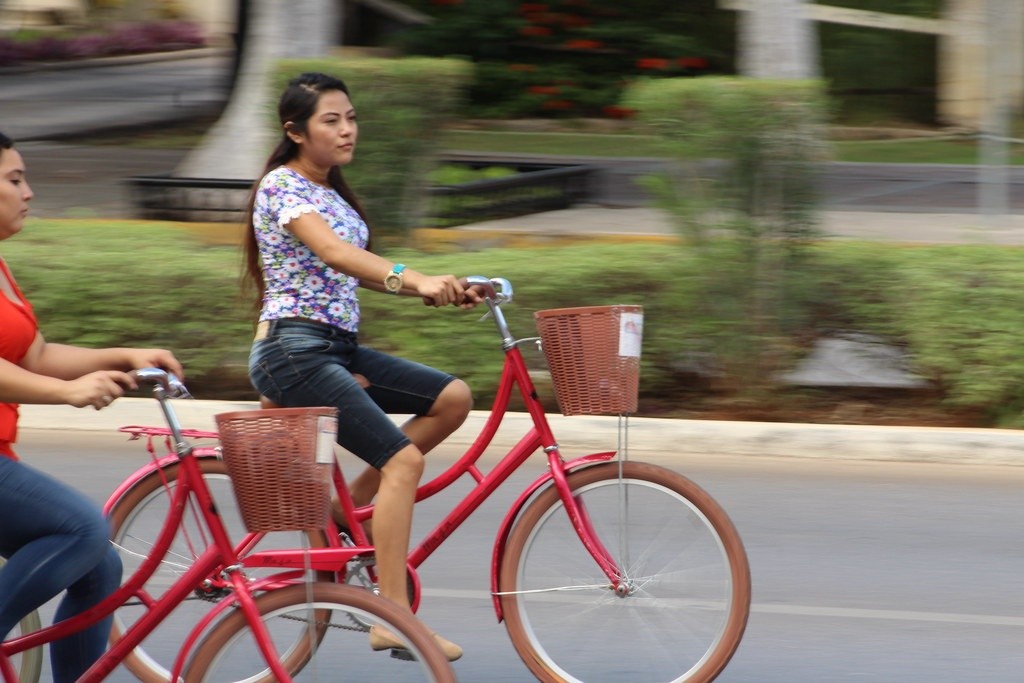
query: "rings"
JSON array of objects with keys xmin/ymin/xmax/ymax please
[{"xmin": 103, "ymin": 395, "xmax": 110, "ymax": 402}]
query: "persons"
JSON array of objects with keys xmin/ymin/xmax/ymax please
[
  {"xmin": 0, "ymin": 132, "xmax": 183, "ymax": 683},
  {"xmin": 239, "ymin": 73, "xmax": 473, "ymax": 661}
]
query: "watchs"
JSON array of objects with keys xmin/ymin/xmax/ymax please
[{"xmin": 384, "ymin": 263, "xmax": 406, "ymax": 294}]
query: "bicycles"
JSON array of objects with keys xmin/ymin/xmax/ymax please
[
  {"xmin": 2, "ymin": 365, "xmax": 463, "ymax": 683},
  {"xmin": 102, "ymin": 275, "xmax": 755, "ymax": 683}
]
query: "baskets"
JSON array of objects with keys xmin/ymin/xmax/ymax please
[
  {"xmin": 533, "ymin": 305, "xmax": 643, "ymax": 416},
  {"xmin": 214, "ymin": 407, "xmax": 339, "ymax": 534}
]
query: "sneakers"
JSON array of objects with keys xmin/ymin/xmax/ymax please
[
  {"xmin": 369, "ymin": 627, "xmax": 463, "ymax": 662},
  {"xmin": 331, "ymin": 497, "xmax": 372, "ymax": 540}
]
